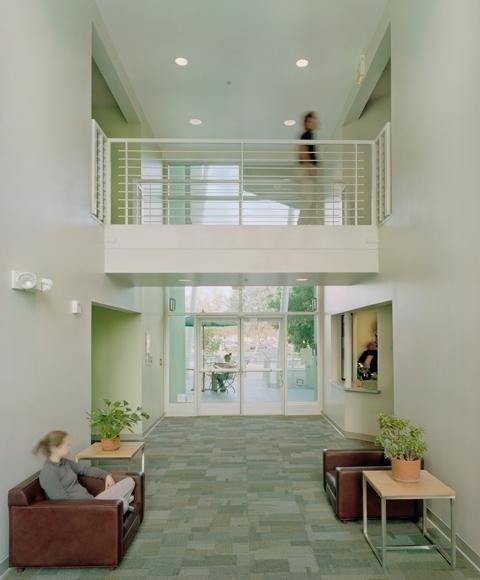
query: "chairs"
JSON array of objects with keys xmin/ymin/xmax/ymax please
[
  {"xmin": 322, "ymin": 447, "xmax": 424, "ymax": 521},
  {"xmin": 8, "ymin": 472, "xmax": 143, "ymax": 568}
]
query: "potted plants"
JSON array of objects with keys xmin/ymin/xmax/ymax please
[
  {"xmin": 87, "ymin": 399, "xmax": 149, "ymax": 450},
  {"xmin": 374, "ymin": 414, "xmax": 426, "ymax": 481}
]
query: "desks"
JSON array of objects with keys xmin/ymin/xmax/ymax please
[
  {"xmin": 76, "ymin": 441, "xmax": 146, "ymax": 474},
  {"xmin": 363, "ymin": 469, "xmax": 457, "ymax": 570}
]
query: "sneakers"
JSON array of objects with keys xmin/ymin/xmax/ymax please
[{"xmin": 213, "ymin": 390, "xmax": 225, "ymax": 392}]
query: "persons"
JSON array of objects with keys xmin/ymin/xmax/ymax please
[
  {"xmin": 211, "ymin": 352, "xmax": 232, "ymax": 393},
  {"xmin": 358, "ymin": 341, "xmax": 377, "ymax": 382},
  {"xmin": 293, "ymin": 110, "xmax": 326, "ymax": 225},
  {"xmin": 33, "ymin": 430, "xmax": 137, "ymax": 520}
]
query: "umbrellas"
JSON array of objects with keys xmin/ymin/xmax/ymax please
[{"xmin": 186, "ymin": 309, "xmax": 238, "ymax": 390}]
